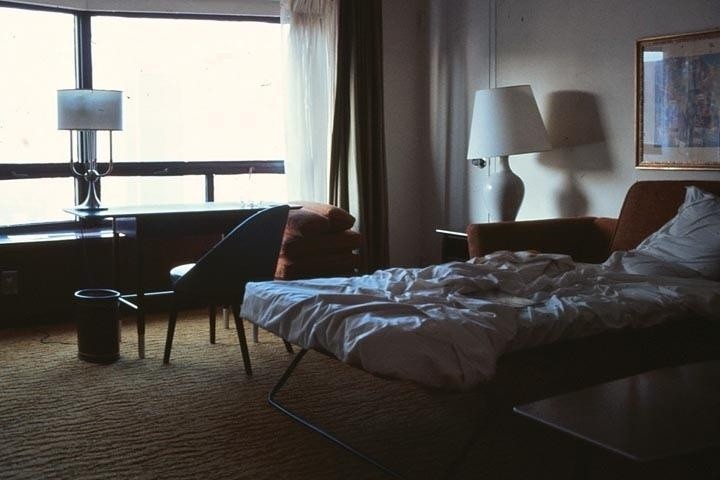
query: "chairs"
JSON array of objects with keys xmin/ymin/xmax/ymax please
[{"xmin": 163, "ymin": 204, "xmax": 296, "ymax": 377}]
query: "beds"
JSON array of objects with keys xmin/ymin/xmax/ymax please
[{"xmin": 241, "ymin": 181, "xmax": 720, "ymax": 477}]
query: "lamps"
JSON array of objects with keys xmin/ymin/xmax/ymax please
[
  {"xmin": 465, "ymin": 84, "xmax": 553, "ymax": 223},
  {"xmin": 56, "ymin": 88, "xmax": 125, "ymax": 211}
]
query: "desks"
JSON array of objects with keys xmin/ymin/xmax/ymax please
[
  {"xmin": 63, "ymin": 201, "xmax": 300, "ymax": 364},
  {"xmin": 514, "ymin": 360, "xmax": 720, "ymax": 479}
]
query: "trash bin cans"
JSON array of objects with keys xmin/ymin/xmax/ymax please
[{"xmin": 74, "ymin": 288, "xmax": 121, "ymax": 363}]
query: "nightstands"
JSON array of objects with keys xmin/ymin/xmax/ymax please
[{"xmin": 434, "ymin": 226, "xmax": 470, "ymax": 264}]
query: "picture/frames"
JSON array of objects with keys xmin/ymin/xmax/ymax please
[{"xmin": 633, "ymin": 27, "xmax": 719, "ymax": 172}]
